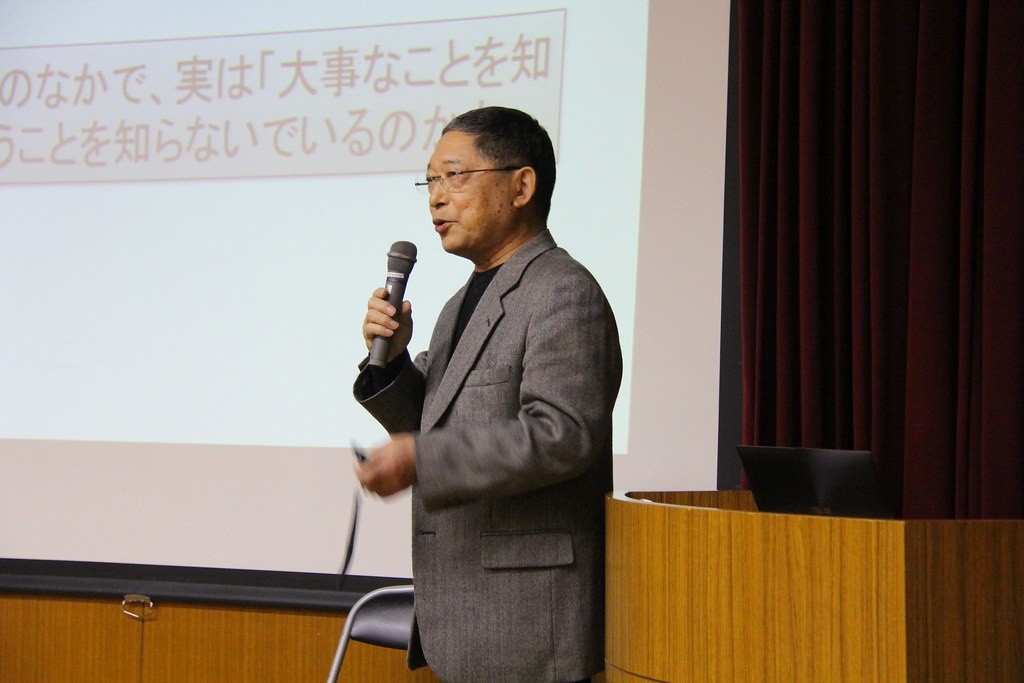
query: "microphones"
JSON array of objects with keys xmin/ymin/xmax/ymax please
[{"xmin": 368, "ymin": 241, "xmax": 417, "ymax": 369}]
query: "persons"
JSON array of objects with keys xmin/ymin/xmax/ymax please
[{"xmin": 352, "ymin": 106, "xmax": 623, "ymax": 683}]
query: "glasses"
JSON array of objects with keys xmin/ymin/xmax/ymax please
[{"xmin": 415, "ymin": 165, "xmax": 521, "ymax": 194}]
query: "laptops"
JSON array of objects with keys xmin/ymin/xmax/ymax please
[{"xmin": 736, "ymin": 445, "xmax": 895, "ymax": 522}]
